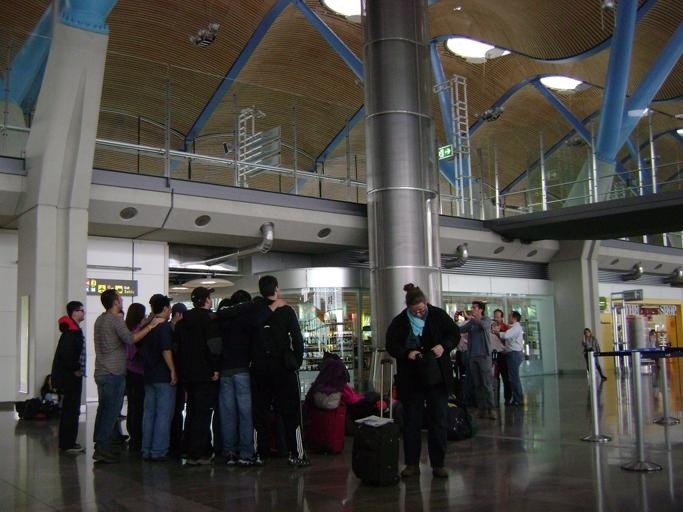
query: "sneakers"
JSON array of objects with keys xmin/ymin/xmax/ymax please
[
  {"xmin": 227, "ymin": 456, "xmax": 237, "ymax": 464},
  {"xmin": 289, "ymin": 457, "xmax": 311, "ymax": 467},
  {"xmin": 64, "ymin": 445, "xmax": 84, "ymax": 452},
  {"xmin": 238, "ymin": 456, "xmax": 264, "ymax": 464},
  {"xmin": 489, "ymin": 410, "xmax": 496, "ymax": 419},
  {"xmin": 188, "ymin": 455, "xmax": 213, "ymax": 464},
  {"xmin": 93, "ymin": 452, "xmax": 120, "ymax": 464},
  {"xmin": 401, "ymin": 466, "xmax": 419, "ymax": 476},
  {"xmin": 433, "ymin": 466, "xmax": 448, "ymax": 478}
]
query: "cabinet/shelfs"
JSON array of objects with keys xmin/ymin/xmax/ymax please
[{"xmin": 298, "ymin": 303, "xmax": 354, "ymax": 371}]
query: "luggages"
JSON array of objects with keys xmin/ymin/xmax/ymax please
[
  {"xmin": 304, "ymin": 399, "xmax": 346, "ymax": 454},
  {"xmin": 352, "ymin": 358, "xmax": 399, "ymax": 480},
  {"xmin": 346, "ymin": 348, "xmax": 388, "ymax": 433}
]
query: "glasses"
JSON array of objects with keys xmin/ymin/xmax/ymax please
[
  {"xmin": 412, "ymin": 308, "xmax": 425, "ymax": 315},
  {"xmin": 76, "ymin": 309, "xmax": 84, "ymax": 311}
]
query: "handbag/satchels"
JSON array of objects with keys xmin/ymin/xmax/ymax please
[
  {"xmin": 314, "ymin": 352, "xmax": 349, "ymax": 392},
  {"xmin": 448, "ymin": 402, "xmax": 472, "ymax": 441},
  {"xmin": 416, "ymin": 351, "xmax": 443, "ymax": 389}
]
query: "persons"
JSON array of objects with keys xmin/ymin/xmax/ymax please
[
  {"xmin": 649, "ymin": 329, "xmax": 659, "ymax": 348},
  {"xmin": 454, "ymin": 301, "xmax": 525, "ymax": 420},
  {"xmin": 583, "ymin": 327, "xmax": 608, "ymax": 380},
  {"xmin": 93, "ymin": 275, "xmax": 311, "ymax": 467},
  {"xmin": 51, "ymin": 301, "xmax": 86, "ymax": 453},
  {"xmin": 40, "ymin": 375, "xmax": 61, "ymax": 420},
  {"xmin": 385, "ymin": 282, "xmax": 461, "ymax": 478}
]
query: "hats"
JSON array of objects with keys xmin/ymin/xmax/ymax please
[
  {"xmin": 149, "ymin": 294, "xmax": 173, "ymax": 303},
  {"xmin": 191, "ymin": 287, "xmax": 215, "ymax": 302}
]
